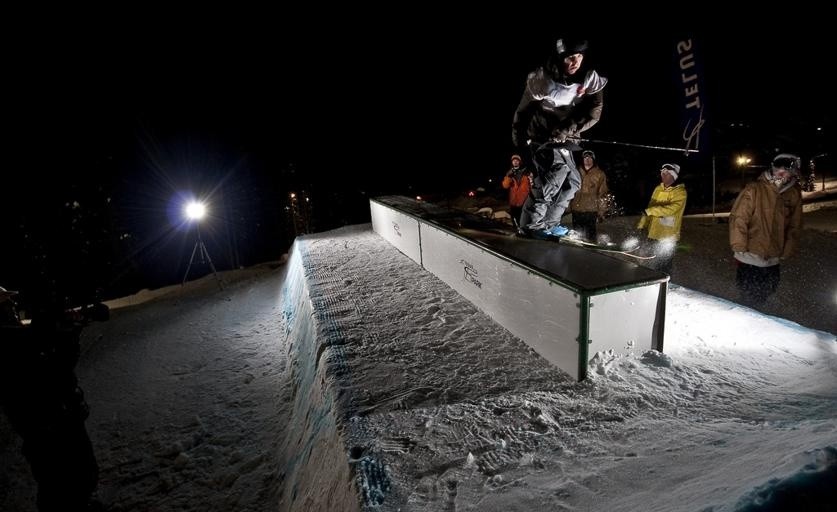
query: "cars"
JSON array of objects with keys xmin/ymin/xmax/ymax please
[{"xmin": 800, "ymin": 182, "xmax": 814, "ymax": 191}]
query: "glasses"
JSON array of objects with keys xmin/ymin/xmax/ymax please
[{"xmin": 584, "ymin": 152, "xmax": 593, "ymax": 158}]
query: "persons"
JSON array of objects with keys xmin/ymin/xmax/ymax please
[
  {"xmin": 1, "ymin": 285, "xmax": 111, "ymax": 511},
  {"xmin": 502, "ymin": 154, "xmax": 533, "ymax": 228},
  {"xmin": 728, "ymin": 151, "xmax": 803, "ymax": 305},
  {"xmin": 564, "ymin": 149, "xmax": 608, "ymax": 246},
  {"xmin": 510, "ymin": 35, "xmax": 610, "ymax": 231},
  {"xmin": 635, "ymin": 161, "xmax": 689, "ymax": 274}
]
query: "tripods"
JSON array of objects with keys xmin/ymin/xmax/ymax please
[{"xmin": 182, "ymin": 221, "xmax": 225, "ymax": 291}]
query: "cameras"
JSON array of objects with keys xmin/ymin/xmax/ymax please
[{"xmin": 512, "ymin": 167, "xmax": 519, "ymax": 175}]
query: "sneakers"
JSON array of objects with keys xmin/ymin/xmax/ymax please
[{"xmin": 533, "ymin": 226, "xmax": 569, "ymax": 237}]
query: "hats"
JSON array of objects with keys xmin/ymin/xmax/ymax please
[
  {"xmin": 582, "ymin": 150, "xmax": 596, "ymax": 160},
  {"xmin": 556, "ymin": 32, "xmax": 588, "ymax": 54},
  {"xmin": 511, "ymin": 155, "xmax": 521, "ymax": 161},
  {"xmin": 661, "ymin": 163, "xmax": 680, "ymax": 179}
]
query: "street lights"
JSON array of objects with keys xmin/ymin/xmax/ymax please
[
  {"xmin": 735, "ymin": 153, "xmax": 752, "ymax": 181},
  {"xmin": 288, "ymin": 190, "xmax": 311, "ymax": 234}
]
